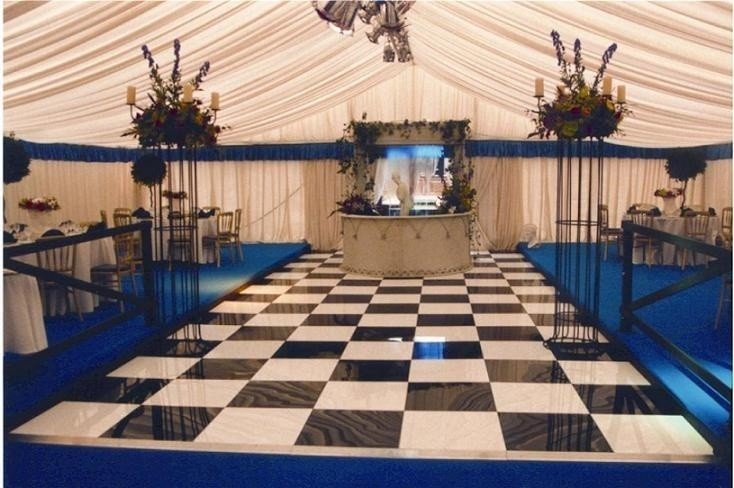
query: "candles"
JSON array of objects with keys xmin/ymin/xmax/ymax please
[
  {"xmin": 184, "ymin": 81, "xmax": 194, "ymax": 102},
  {"xmin": 534, "ymin": 76, "xmax": 545, "ymax": 96},
  {"xmin": 126, "ymin": 85, "xmax": 137, "ymax": 103},
  {"xmin": 617, "ymin": 85, "xmax": 628, "ymax": 104},
  {"xmin": 557, "ymin": 84, "xmax": 565, "ymax": 95},
  {"xmin": 602, "ymin": 75, "xmax": 613, "ymax": 95},
  {"xmin": 210, "ymin": 91, "xmax": 219, "ymax": 109}
]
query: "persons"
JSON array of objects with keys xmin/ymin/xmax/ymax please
[{"xmin": 390, "ymin": 170, "xmax": 414, "ymax": 216}]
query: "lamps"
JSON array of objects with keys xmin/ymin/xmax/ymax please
[{"xmin": 313, "ymin": 0, "xmax": 419, "ymax": 64}]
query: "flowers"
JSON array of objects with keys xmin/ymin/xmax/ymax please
[
  {"xmin": 524, "ymin": 28, "xmax": 633, "ymax": 146},
  {"xmin": 17, "ymin": 196, "xmax": 60, "ymax": 212},
  {"xmin": 435, "ymin": 157, "xmax": 479, "ymax": 214},
  {"xmin": 121, "ymin": 40, "xmax": 232, "ymax": 149},
  {"xmin": 162, "ymin": 189, "xmax": 187, "ymax": 200},
  {"xmin": 330, "ymin": 191, "xmax": 384, "ymax": 218},
  {"xmin": 655, "ymin": 187, "xmax": 684, "ymax": 215}
]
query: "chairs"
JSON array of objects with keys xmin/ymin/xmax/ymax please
[
  {"xmin": 599, "ymin": 203, "xmax": 733, "ymax": 268},
  {"xmin": 3, "ymin": 204, "xmax": 241, "ymax": 324}
]
